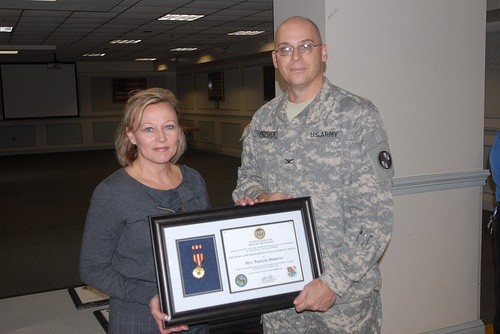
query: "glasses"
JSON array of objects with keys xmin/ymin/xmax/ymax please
[{"xmin": 276, "ymin": 42, "xmax": 322, "ymax": 56}]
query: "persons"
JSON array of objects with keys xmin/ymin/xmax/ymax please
[
  {"xmin": 80, "ymin": 88, "xmax": 266, "ymax": 334},
  {"xmin": 488, "ymin": 131, "xmax": 500, "ymax": 334},
  {"xmin": 233, "ymin": 16, "xmax": 394, "ymax": 334}
]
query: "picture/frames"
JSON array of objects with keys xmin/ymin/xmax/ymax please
[
  {"xmin": 147, "ymin": 195, "xmax": 323, "ymax": 330},
  {"xmin": 67, "ymin": 285, "xmax": 111, "ymax": 311}
]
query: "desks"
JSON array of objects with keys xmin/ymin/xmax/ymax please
[{"xmin": 0, "ymin": 289, "xmax": 110, "ymax": 334}]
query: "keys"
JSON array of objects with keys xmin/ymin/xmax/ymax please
[{"xmin": 487, "ymin": 215, "xmax": 495, "ymax": 234}]
